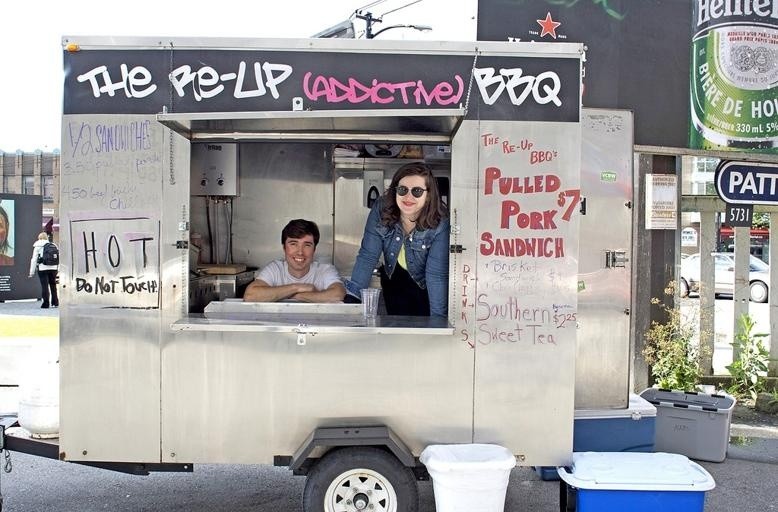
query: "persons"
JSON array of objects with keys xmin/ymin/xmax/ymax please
[
  {"xmin": 28, "ymin": 231, "xmax": 60, "ymax": 309},
  {"xmin": 341, "ymin": 163, "xmax": 450, "ymax": 319},
  {"xmin": 243, "ymin": 217, "xmax": 348, "ymax": 302},
  {"xmin": 0, "ymin": 207, "xmax": 14, "ymax": 265}
]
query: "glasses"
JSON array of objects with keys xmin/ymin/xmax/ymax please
[{"xmin": 394, "ymin": 185, "xmax": 427, "ymax": 198}]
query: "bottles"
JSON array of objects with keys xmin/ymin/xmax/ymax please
[{"xmin": 191, "ymin": 232, "xmax": 201, "ymax": 264}]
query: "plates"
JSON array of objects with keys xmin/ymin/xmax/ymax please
[{"xmin": 365, "ymin": 144, "xmax": 404, "ymax": 158}]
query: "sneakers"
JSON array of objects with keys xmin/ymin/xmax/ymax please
[{"xmin": 41, "ymin": 303, "xmax": 58, "ymax": 308}]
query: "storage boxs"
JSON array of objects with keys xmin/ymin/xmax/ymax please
[{"xmin": 534, "ymin": 386, "xmax": 739, "ymax": 512}]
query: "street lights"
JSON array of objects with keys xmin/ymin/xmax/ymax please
[{"xmin": 364, "ymin": 11, "xmax": 432, "ymax": 40}]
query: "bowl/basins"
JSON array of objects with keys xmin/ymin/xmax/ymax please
[{"xmin": 18, "ymin": 400, "xmax": 59, "ymax": 439}]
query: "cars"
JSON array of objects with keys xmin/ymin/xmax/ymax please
[{"xmin": 680, "ymin": 252, "xmax": 769, "ymax": 303}]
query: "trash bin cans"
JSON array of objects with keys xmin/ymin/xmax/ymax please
[{"xmin": 419, "ymin": 444, "xmax": 516, "ymax": 512}]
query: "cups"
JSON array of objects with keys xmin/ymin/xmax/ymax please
[{"xmin": 360, "ymin": 289, "xmax": 380, "ymax": 319}]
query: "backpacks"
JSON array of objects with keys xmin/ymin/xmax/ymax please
[{"xmin": 38, "ymin": 242, "xmax": 59, "ymax": 265}]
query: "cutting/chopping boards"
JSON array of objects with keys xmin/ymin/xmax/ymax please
[{"xmin": 195, "ymin": 263, "xmax": 247, "ymax": 274}]
